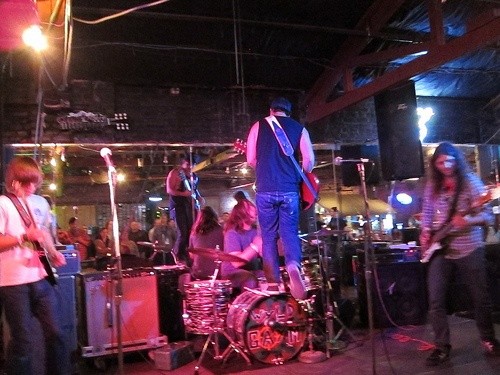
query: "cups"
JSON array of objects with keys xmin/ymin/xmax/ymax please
[{"xmin": 402, "ymin": 228, "xmax": 419, "ymax": 246}]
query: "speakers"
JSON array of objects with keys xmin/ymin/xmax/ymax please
[
  {"xmin": 1, "ymin": 274, "xmax": 76, "ymax": 363},
  {"xmin": 375, "ymin": 80, "xmax": 425, "ymax": 180},
  {"xmin": 75, "ymin": 268, "xmax": 159, "ymax": 346},
  {"xmin": 371, "ymin": 261, "xmax": 428, "ymax": 329}
]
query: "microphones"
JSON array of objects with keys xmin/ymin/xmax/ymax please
[
  {"xmin": 267, "ymin": 318, "xmax": 279, "ymax": 328},
  {"xmin": 100, "ymin": 147, "xmax": 116, "ymax": 175},
  {"xmin": 106, "ymin": 301, "xmax": 112, "ymax": 327},
  {"xmin": 334, "ymin": 157, "xmax": 373, "ymax": 165}
]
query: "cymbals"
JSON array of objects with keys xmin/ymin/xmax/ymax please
[
  {"xmin": 186, "ymin": 247, "xmax": 247, "ymax": 262},
  {"xmin": 299, "ymin": 227, "xmax": 353, "ymax": 237}
]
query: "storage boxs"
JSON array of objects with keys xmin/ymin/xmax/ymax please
[{"xmin": 155, "ymin": 340, "xmax": 194, "ymax": 370}]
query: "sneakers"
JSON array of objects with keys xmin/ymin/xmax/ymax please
[
  {"xmin": 426, "ymin": 343, "xmax": 452, "ymax": 366},
  {"xmin": 479, "ymin": 339, "xmax": 500, "ymax": 359}
]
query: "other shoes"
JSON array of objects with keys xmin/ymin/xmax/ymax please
[
  {"xmin": 286, "ymin": 264, "xmax": 308, "ymax": 299},
  {"xmin": 266, "ymin": 286, "xmax": 279, "ymax": 291},
  {"xmin": 170, "ymin": 251, "xmax": 180, "ymax": 265}
]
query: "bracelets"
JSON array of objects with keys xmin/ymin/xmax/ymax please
[{"xmin": 22, "ymin": 234, "xmax": 28, "ymax": 241}]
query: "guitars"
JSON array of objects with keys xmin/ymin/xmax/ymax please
[
  {"xmin": 419, "ymin": 189, "xmax": 493, "ymax": 263},
  {"xmin": 177, "ymin": 170, "xmax": 202, "ymax": 211},
  {"xmin": 12, "ymin": 180, "xmax": 58, "ymax": 287},
  {"xmin": 233, "ymin": 137, "xmax": 320, "ymax": 211}
]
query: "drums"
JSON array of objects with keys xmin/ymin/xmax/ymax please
[
  {"xmin": 280, "ymin": 259, "xmax": 325, "ymax": 294},
  {"xmin": 226, "ymin": 287, "xmax": 308, "ymax": 364},
  {"xmin": 182, "ymin": 279, "xmax": 233, "ymax": 334}
]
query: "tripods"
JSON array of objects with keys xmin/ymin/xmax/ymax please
[{"xmin": 194, "ymin": 258, "xmax": 251, "ymax": 375}]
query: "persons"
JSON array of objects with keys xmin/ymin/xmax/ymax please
[
  {"xmin": 42, "ymin": 152, "xmax": 266, "ymax": 292},
  {"xmin": 327, "ymin": 207, "xmax": 346, "ymax": 231},
  {"xmin": 0, "ymin": 155, "xmax": 71, "ymax": 375},
  {"xmin": 246, "ymin": 97, "xmax": 315, "ymax": 300},
  {"xmin": 419, "ymin": 141, "xmax": 500, "ymax": 366}
]
